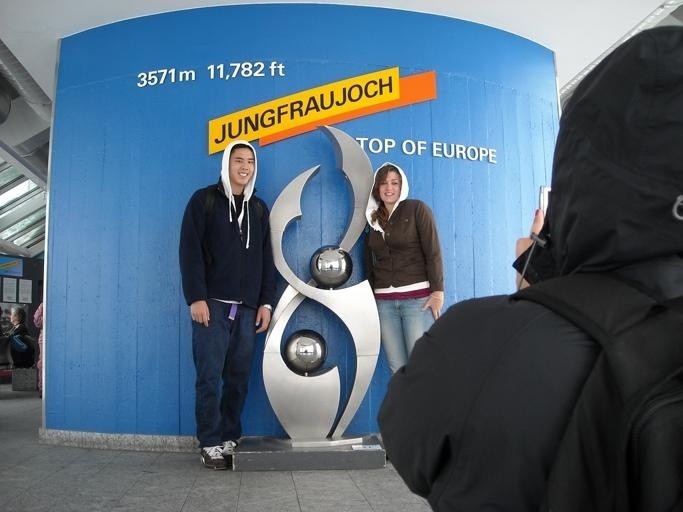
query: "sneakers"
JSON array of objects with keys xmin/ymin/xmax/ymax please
[{"xmin": 200, "ymin": 441, "xmax": 239, "ymax": 472}]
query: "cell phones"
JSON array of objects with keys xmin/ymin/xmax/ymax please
[{"xmin": 538, "ymin": 180, "xmax": 552, "ymax": 223}]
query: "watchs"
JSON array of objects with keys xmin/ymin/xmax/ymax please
[{"xmin": 260, "ymin": 304, "xmax": 272, "ymax": 311}]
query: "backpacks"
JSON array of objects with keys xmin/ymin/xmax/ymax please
[{"xmin": 539, "ymin": 290, "xmax": 683, "ymax": 510}]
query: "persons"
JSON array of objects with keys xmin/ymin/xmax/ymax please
[
  {"xmin": 378, "ymin": 26, "xmax": 683, "ymax": 512},
  {"xmin": 179, "ymin": 141, "xmax": 277, "ymax": 469},
  {"xmin": 0, "ymin": 306, "xmax": 28, "ymax": 341},
  {"xmin": 365, "ymin": 162, "xmax": 445, "ymax": 374},
  {"xmin": 34, "ymin": 303, "xmax": 43, "ymax": 398}
]
